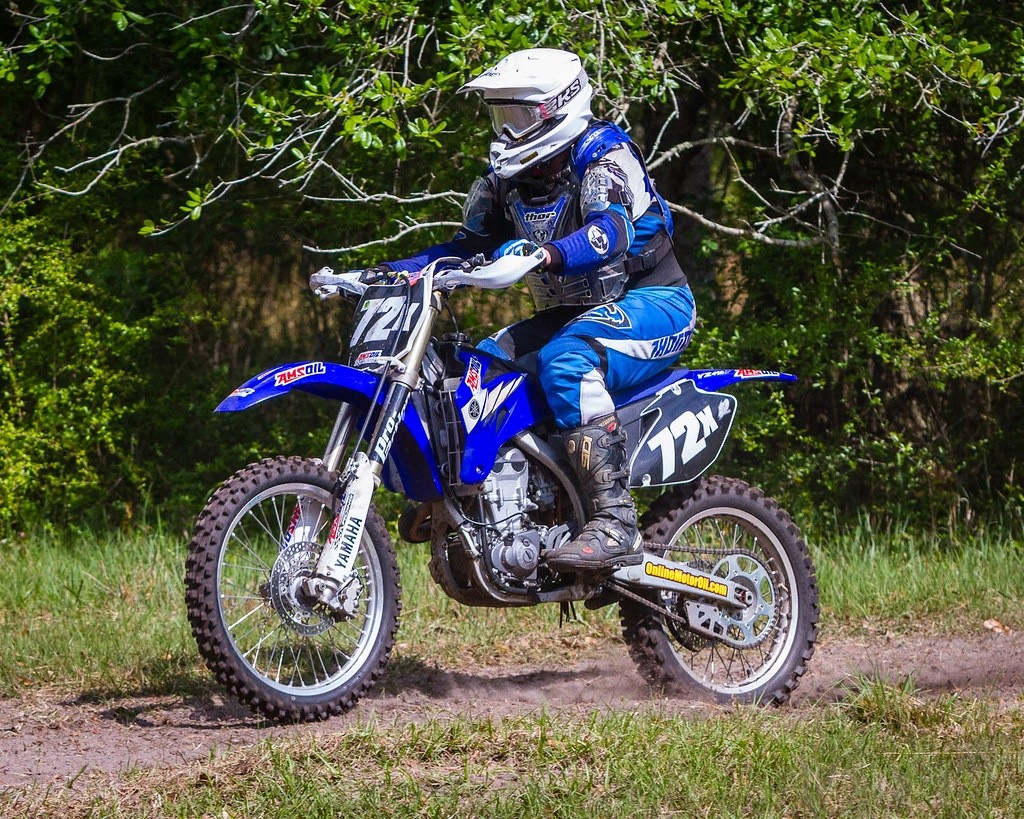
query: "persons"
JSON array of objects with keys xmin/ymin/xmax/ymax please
[{"xmin": 338, "ymin": 46, "xmax": 696, "ymax": 570}]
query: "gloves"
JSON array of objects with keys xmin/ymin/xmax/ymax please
[
  {"xmin": 492, "ymin": 239, "xmax": 547, "ymax": 274},
  {"xmin": 339, "ymin": 269, "xmax": 384, "ymax": 302}
]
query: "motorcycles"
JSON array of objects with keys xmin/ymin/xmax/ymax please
[{"xmin": 182, "ymin": 240, "xmax": 822, "ymax": 726}]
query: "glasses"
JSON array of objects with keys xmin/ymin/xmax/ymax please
[{"xmin": 484, "ymin": 99, "xmax": 545, "ymax": 141}]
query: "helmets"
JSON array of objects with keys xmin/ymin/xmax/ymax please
[{"xmin": 455, "ymin": 48, "xmax": 593, "ymax": 179}]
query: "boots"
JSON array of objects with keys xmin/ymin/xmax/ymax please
[{"xmin": 547, "ymin": 412, "xmax": 644, "ymax": 568}]
